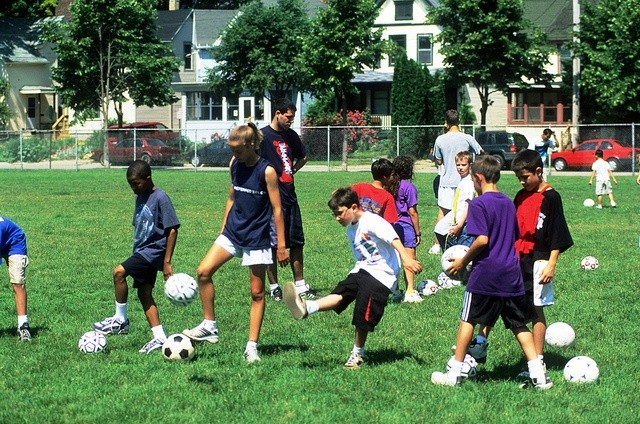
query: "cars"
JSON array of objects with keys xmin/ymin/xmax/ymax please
[
  {"xmin": 546, "ymin": 138, "xmax": 640, "ymax": 171},
  {"xmin": 186, "ymin": 139, "xmax": 233, "ymax": 167},
  {"xmin": 93, "ymin": 138, "xmax": 180, "ymax": 166}
]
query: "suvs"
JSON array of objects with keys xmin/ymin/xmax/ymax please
[
  {"xmin": 108, "ymin": 122, "xmax": 179, "ymax": 150},
  {"xmin": 475, "ymin": 131, "xmax": 529, "ymax": 170}
]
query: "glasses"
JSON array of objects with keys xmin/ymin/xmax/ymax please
[{"xmin": 332, "ymin": 208, "xmax": 349, "ymax": 217}]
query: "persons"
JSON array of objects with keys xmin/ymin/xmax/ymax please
[
  {"xmin": 451, "ymin": 149, "xmax": 574, "ymax": 382},
  {"xmin": 391, "ymin": 153, "xmax": 420, "ymax": 303},
  {"xmin": 331, "ymin": 156, "xmax": 398, "ymax": 227},
  {"xmin": 433, "ymin": 150, "xmax": 473, "ymax": 293},
  {"xmin": 0, "ymin": 213, "xmax": 32, "ymax": 343},
  {"xmin": 426, "ymin": 105, "xmax": 484, "ymax": 255},
  {"xmin": 527, "ymin": 129, "xmax": 561, "ymax": 183},
  {"xmin": 588, "ymin": 149, "xmax": 618, "ymax": 209},
  {"xmin": 257, "ymin": 98, "xmax": 318, "ymax": 303},
  {"xmin": 282, "ymin": 184, "xmax": 424, "ymax": 371},
  {"xmin": 636, "ymin": 154, "xmax": 640, "ymax": 185},
  {"xmin": 92, "ymin": 159, "xmax": 180, "ymax": 354},
  {"xmin": 430, "ymin": 151, "xmax": 553, "ymax": 390},
  {"xmin": 181, "ymin": 121, "xmax": 291, "ymax": 365}
]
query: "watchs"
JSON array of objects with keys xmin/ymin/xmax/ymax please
[{"xmin": 415, "ymin": 231, "xmax": 421, "ymax": 236}]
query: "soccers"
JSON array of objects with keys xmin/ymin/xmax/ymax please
[
  {"xmin": 162, "ymin": 334, "xmax": 196, "ymax": 362},
  {"xmin": 79, "ymin": 331, "xmax": 107, "ymax": 354},
  {"xmin": 441, "ymin": 245, "xmax": 473, "ymax": 281},
  {"xmin": 581, "ymin": 256, "xmax": 599, "ymax": 270},
  {"xmin": 437, "ymin": 272, "xmax": 460, "ymax": 288},
  {"xmin": 583, "ymin": 198, "xmax": 594, "ymax": 208},
  {"xmin": 446, "ymin": 353, "xmax": 477, "ymax": 379},
  {"xmin": 564, "ymin": 355, "xmax": 600, "ymax": 383},
  {"xmin": 164, "ymin": 273, "xmax": 198, "ymax": 307},
  {"xmin": 418, "ymin": 280, "xmax": 436, "ymax": 296},
  {"xmin": 545, "ymin": 322, "xmax": 575, "ymax": 349}
]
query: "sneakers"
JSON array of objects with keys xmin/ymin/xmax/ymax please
[
  {"xmin": 596, "ymin": 206, "xmax": 602, "ymax": 209},
  {"xmin": 17, "ymin": 322, "xmax": 31, "ymax": 340},
  {"xmin": 404, "ymin": 291, "xmax": 422, "ymax": 302},
  {"xmin": 183, "ymin": 321, "xmax": 219, "ymax": 343},
  {"xmin": 242, "ymin": 349, "xmax": 261, "ymax": 362},
  {"xmin": 342, "ymin": 351, "xmax": 369, "ymax": 369},
  {"xmin": 516, "ymin": 363, "xmax": 550, "ymax": 381},
  {"xmin": 431, "ymin": 371, "xmax": 461, "ymax": 385},
  {"xmin": 451, "ymin": 338, "xmax": 489, "ymax": 362},
  {"xmin": 428, "ymin": 247, "xmax": 440, "ymax": 253},
  {"xmin": 139, "ymin": 337, "xmax": 164, "ymax": 354},
  {"xmin": 93, "ymin": 317, "xmax": 129, "ymax": 336},
  {"xmin": 611, "ymin": 202, "xmax": 616, "ymax": 208},
  {"xmin": 271, "ymin": 287, "xmax": 283, "ymax": 301},
  {"xmin": 283, "ymin": 282, "xmax": 308, "ymax": 320},
  {"xmin": 519, "ymin": 376, "xmax": 553, "ymax": 390},
  {"xmin": 295, "ymin": 284, "xmax": 320, "ymax": 299}
]
